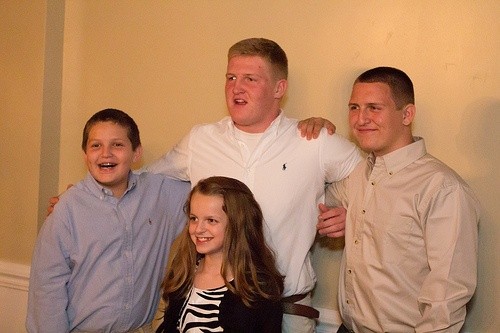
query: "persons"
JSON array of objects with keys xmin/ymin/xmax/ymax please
[
  {"xmin": 298, "ymin": 67, "xmax": 481, "ymax": 333},
  {"xmin": 156, "ymin": 176, "xmax": 284, "ymax": 333},
  {"xmin": 27, "ymin": 110, "xmax": 347, "ymax": 333},
  {"xmin": 46, "ymin": 38, "xmax": 369, "ymax": 333}
]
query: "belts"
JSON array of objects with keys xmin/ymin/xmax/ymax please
[{"xmin": 280, "ymin": 293, "xmax": 319, "ymax": 318}]
